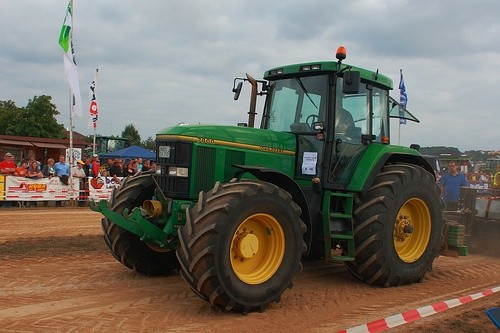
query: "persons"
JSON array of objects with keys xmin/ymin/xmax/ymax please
[
  {"xmin": 439, "ymin": 161, "xmax": 468, "ymax": 203},
  {"xmin": 0, "ymin": 149, "xmax": 156, "ymax": 209},
  {"xmin": 469, "ymin": 171, "xmax": 492, "ymax": 182}
]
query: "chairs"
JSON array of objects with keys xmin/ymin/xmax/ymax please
[{"xmin": 332, "ymin": 108, "xmax": 358, "ymax": 180}]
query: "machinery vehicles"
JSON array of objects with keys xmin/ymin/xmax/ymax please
[{"xmin": 93, "ymin": 46, "xmax": 445, "ymax": 315}]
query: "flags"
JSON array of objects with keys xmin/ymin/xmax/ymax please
[
  {"xmin": 59, "ymin": 0, "xmax": 83, "ymax": 119},
  {"xmin": 398, "ymin": 71, "xmax": 408, "ymax": 126},
  {"xmin": 89, "ymin": 71, "xmax": 99, "ymax": 134}
]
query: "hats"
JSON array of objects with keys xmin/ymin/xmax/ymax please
[
  {"xmin": 77, "ymin": 160, "xmax": 85, "ymax": 165},
  {"xmin": 93, "ymin": 153, "xmax": 98, "ymax": 157}
]
